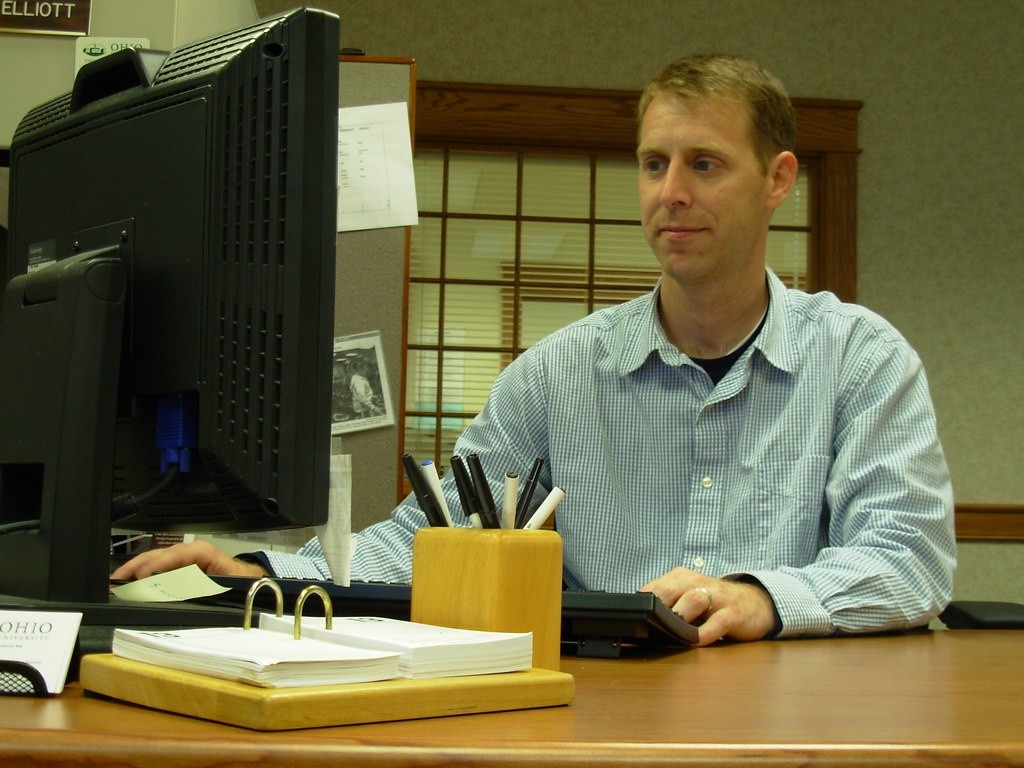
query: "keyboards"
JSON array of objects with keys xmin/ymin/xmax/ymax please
[{"xmin": 192, "ymin": 573, "xmax": 701, "ymax": 659}]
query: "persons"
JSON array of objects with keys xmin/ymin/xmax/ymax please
[
  {"xmin": 110, "ymin": 56, "xmax": 959, "ymax": 646},
  {"xmin": 345, "ymin": 355, "xmax": 382, "ymax": 418}
]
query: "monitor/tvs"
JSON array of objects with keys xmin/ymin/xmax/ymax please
[{"xmin": 0, "ymin": 5, "xmax": 342, "ymax": 662}]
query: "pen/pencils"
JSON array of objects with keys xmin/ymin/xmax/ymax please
[{"xmin": 401, "ymin": 450, "xmax": 566, "ymax": 529}]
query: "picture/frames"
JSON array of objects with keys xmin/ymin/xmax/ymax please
[{"xmin": 331, "ymin": 328, "xmax": 394, "ymax": 435}]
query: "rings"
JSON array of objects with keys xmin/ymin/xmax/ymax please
[{"xmin": 696, "ymin": 588, "xmax": 711, "ymax": 612}]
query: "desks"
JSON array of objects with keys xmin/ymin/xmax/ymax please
[{"xmin": 0, "ymin": 629, "xmax": 1024, "ymax": 768}]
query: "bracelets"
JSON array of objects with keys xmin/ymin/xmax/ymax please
[{"xmin": 372, "ymin": 406, "xmax": 375, "ymax": 410}]
